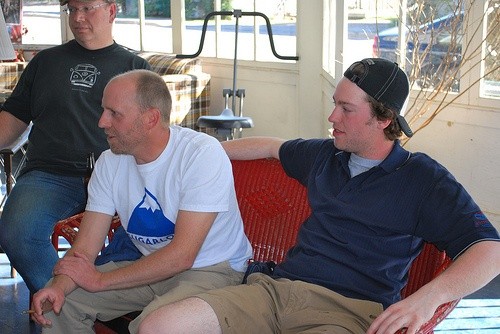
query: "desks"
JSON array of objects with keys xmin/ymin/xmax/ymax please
[{"xmin": 0, "ymin": 123, "xmax": 33, "ymax": 279}]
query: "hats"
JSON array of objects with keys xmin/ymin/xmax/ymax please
[{"xmin": 342, "ymin": 57, "xmax": 415, "ymax": 138}]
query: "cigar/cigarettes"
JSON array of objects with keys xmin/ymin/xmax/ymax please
[{"xmin": 21, "ymin": 309, "xmax": 45, "ymax": 315}]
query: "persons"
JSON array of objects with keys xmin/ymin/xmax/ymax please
[
  {"xmin": 0, "ymin": 0, "xmax": 154, "ymax": 295},
  {"xmin": 137, "ymin": 58, "xmax": 500, "ymax": 334},
  {"xmin": 30, "ymin": 69, "xmax": 253, "ymax": 334}
]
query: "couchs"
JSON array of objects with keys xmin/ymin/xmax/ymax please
[{"xmin": 0, "ymin": 49, "xmax": 211, "ymax": 135}]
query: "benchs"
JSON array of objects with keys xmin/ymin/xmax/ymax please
[{"xmin": 50, "ymin": 157, "xmax": 462, "ymax": 334}]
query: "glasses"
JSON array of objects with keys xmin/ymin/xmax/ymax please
[{"xmin": 63, "ymin": 2, "xmax": 110, "ymax": 14}]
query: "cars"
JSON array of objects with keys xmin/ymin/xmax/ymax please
[{"xmin": 372, "ymin": 11, "xmax": 464, "ymax": 93}]
